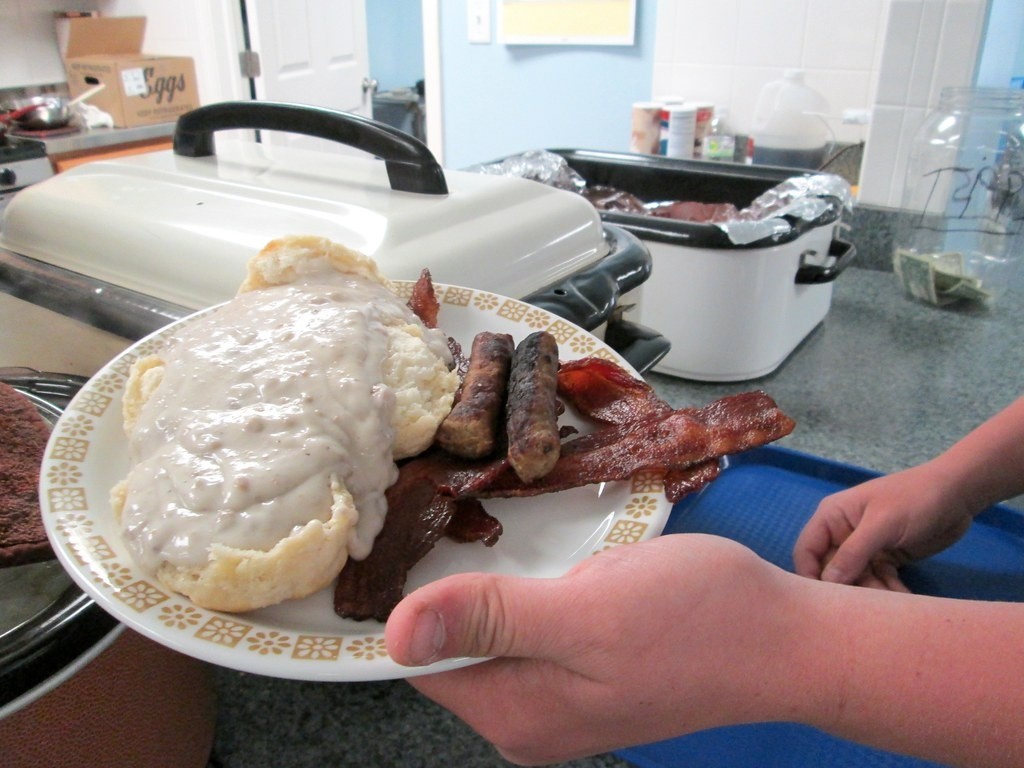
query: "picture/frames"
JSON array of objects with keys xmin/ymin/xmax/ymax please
[{"xmin": 496, "ymin": 0, "xmax": 638, "ymax": 46}]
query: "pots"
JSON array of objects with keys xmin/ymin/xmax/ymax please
[
  {"xmin": 0, "ymin": 363, "xmax": 219, "ymax": 768},
  {"xmin": 462, "ymin": 148, "xmax": 859, "ymax": 382},
  {"xmin": 0, "ymin": 97, "xmax": 672, "ymax": 393},
  {"xmin": 2, "ymin": 95, "xmax": 77, "ymax": 129}
]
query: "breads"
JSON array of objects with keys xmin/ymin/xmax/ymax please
[
  {"xmin": 0, "ymin": 381, "xmax": 63, "ymax": 568},
  {"xmin": 99, "ymin": 233, "xmax": 459, "ymax": 612}
]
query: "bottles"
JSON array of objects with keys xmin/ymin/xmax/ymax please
[{"xmin": 701, "ymin": 104, "xmax": 736, "ymax": 163}]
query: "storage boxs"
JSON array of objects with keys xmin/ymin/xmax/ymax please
[
  {"xmin": 65, "ymin": 15, "xmax": 200, "ymax": 128},
  {"xmin": 456, "ymin": 149, "xmax": 857, "ymax": 383}
]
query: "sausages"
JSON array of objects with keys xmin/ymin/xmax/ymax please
[{"xmin": 433, "ymin": 329, "xmax": 561, "ymax": 484}]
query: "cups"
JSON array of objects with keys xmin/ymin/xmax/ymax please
[
  {"xmin": 666, "ymin": 106, "xmax": 696, "ymax": 160},
  {"xmin": 631, "ymin": 102, "xmax": 662, "ymax": 154}
]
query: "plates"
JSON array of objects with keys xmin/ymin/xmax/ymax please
[{"xmin": 37, "ymin": 280, "xmax": 675, "ymax": 684}]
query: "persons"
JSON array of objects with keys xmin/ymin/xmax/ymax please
[{"xmin": 383, "ymin": 393, "xmax": 1024, "ymax": 768}]
